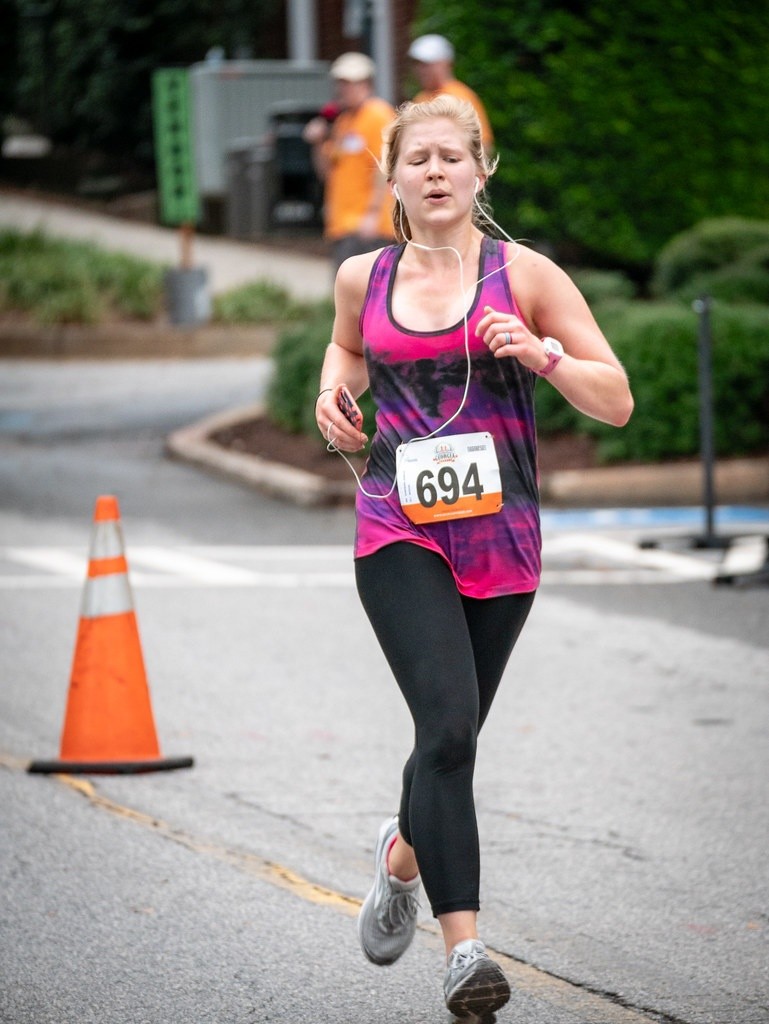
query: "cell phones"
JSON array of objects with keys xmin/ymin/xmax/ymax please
[{"xmin": 337, "ymin": 386, "xmax": 363, "ymax": 432}]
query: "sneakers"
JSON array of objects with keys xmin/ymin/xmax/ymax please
[
  {"xmin": 442, "ymin": 939, "xmax": 510, "ymax": 1024},
  {"xmin": 356, "ymin": 815, "xmax": 421, "ymax": 967}
]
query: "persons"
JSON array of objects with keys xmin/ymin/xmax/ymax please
[
  {"xmin": 316, "ymin": 94, "xmax": 634, "ymax": 1022},
  {"xmin": 302, "ymin": 32, "xmax": 494, "ymax": 275}
]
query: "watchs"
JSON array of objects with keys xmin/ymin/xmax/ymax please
[{"xmin": 535, "ymin": 335, "xmax": 565, "ymax": 379}]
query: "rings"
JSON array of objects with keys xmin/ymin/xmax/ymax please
[{"xmin": 505, "ymin": 332, "xmax": 513, "ymax": 344}]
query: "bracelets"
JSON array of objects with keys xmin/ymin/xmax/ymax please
[{"xmin": 314, "ymin": 388, "xmax": 334, "ymax": 412}]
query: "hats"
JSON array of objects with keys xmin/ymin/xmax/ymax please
[
  {"xmin": 327, "ymin": 51, "xmax": 377, "ymax": 81},
  {"xmin": 404, "ymin": 34, "xmax": 453, "ymax": 63}
]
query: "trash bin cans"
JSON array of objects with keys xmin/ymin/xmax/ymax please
[
  {"xmin": 268, "ymin": 101, "xmax": 329, "ymax": 238},
  {"xmin": 224, "ymin": 134, "xmax": 275, "ymax": 242}
]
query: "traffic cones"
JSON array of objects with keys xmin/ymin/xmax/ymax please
[{"xmin": 23, "ymin": 494, "xmax": 194, "ymax": 775}]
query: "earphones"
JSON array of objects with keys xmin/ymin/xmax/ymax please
[
  {"xmin": 474, "ymin": 176, "xmax": 479, "ymax": 193},
  {"xmin": 393, "ymin": 183, "xmax": 400, "ymax": 201}
]
